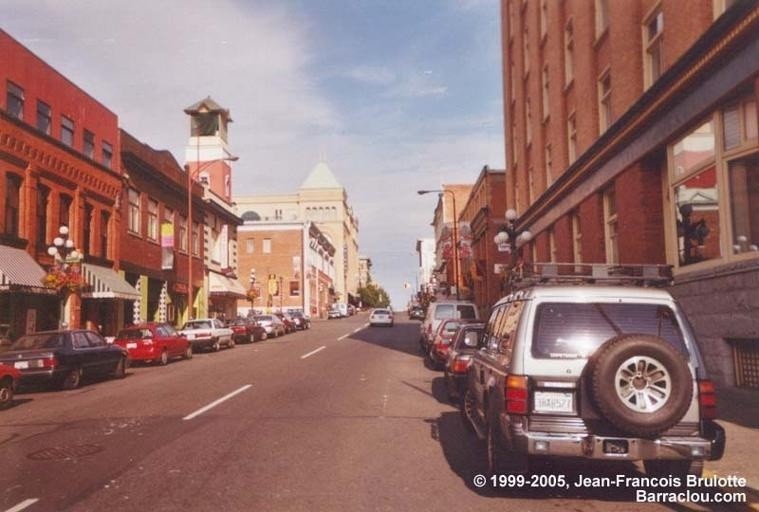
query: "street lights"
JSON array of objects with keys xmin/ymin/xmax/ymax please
[
  {"xmin": 249, "ymin": 268, "xmax": 256, "ymax": 318},
  {"xmin": 497, "ymin": 209, "xmax": 531, "ymax": 280},
  {"xmin": 420, "ymin": 189, "xmax": 461, "ymax": 301},
  {"xmin": 187, "ymin": 157, "xmax": 237, "ymax": 320},
  {"xmin": 47, "ymin": 226, "xmax": 80, "ymax": 329}
]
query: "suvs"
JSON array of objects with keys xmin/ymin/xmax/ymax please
[{"xmin": 464, "ymin": 263, "xmax": 726, "ymax": 490}]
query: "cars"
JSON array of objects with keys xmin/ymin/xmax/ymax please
[
  {"xmin": 1, "ymin": 330, "xmax": 129, "ymax": 390},
  {"xmin": 408, "ymin": 305, "xmax": 425, "ymax": 319},
  {"xmin": 113, "ymin": 321, "xmax": 193, "ymax": 366},
  {"xmin": 426, "ymin": 319, "xmax": 468, "ymax": 371},
  {"xmin": 347, "ymin": 304, "xmax": 355, "ymax": 315},
  {"xmin": 178, "ymin": 318, "xmax": 233, "ymax": 351},
  {"xmin": 330, "ymin": 303, "xmax": 349, "ymax": 318},
  {"xmin": 277, "ymin": 312, "xmax": 296, "ymax": 332},
  {"xmin": 443, "ymin": 324, "xmax": 488, "ymax": 399},
  {"xmin": 289, "ymin": 311, "xmax": 311, "ymax": 330},
  {"xmin": 0, "ymin": 364, "xmax": 23, "ymax": 409},
  {"xmin": 369, "ymin": 309, "xmax": 393, "ymax": 326},
  {"xmin": 229, "ymin": 318, "xmax": 267, "ymax": 342},
  {"xmin": 253, "ymin": 315, "xmax": 286, "ymax": 336}
]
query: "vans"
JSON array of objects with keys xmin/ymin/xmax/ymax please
[{"xmin": 419, "ymin": 301, "xmax": 480, "ymax": 351}]
